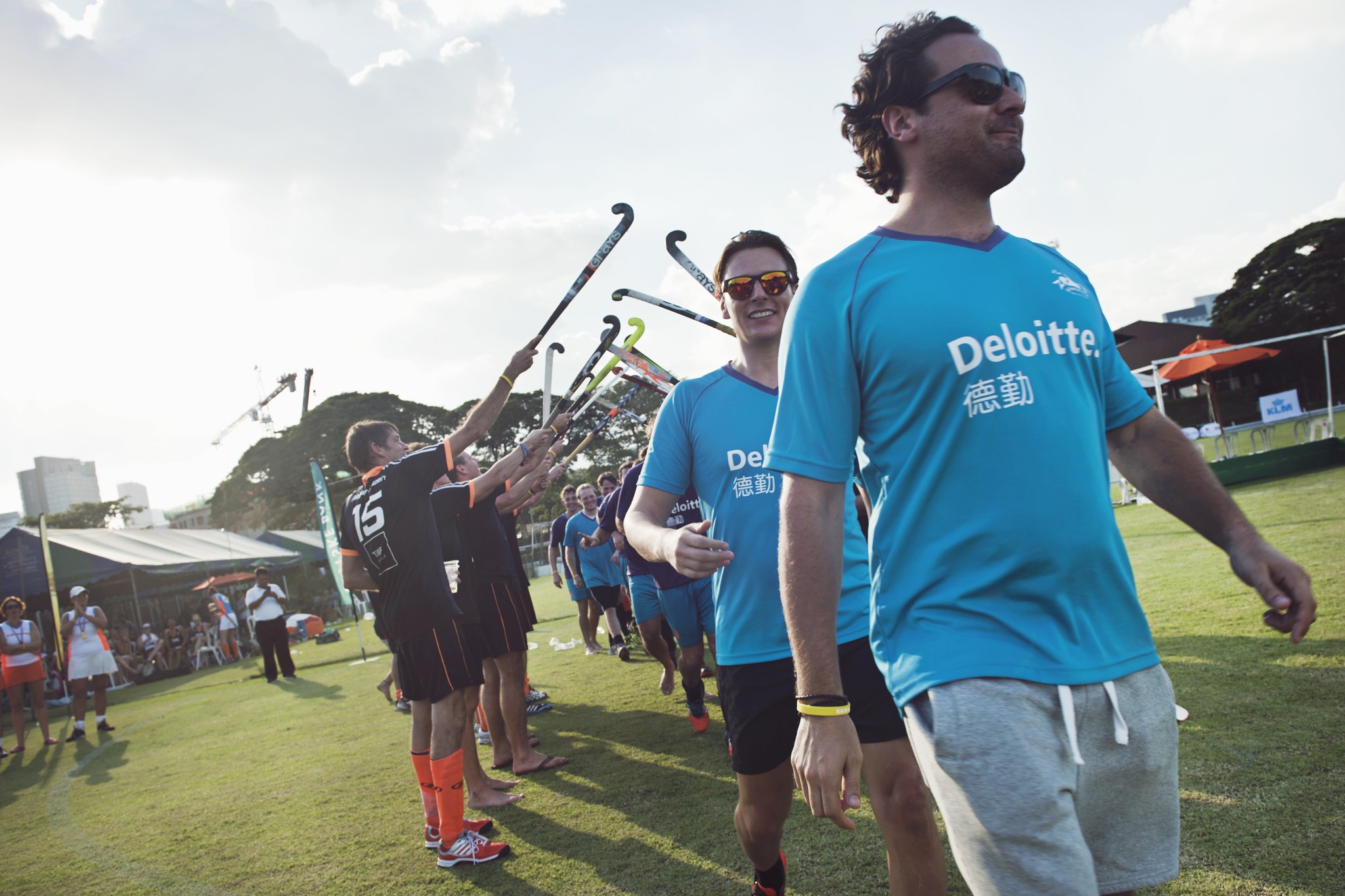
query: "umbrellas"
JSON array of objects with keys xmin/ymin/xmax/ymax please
[
  {"xmin": 1127, "ymin": 372, "xmax": 1170, "ymax": 390},
  {"xmin": 1150, "ymin": 334, "xmax": 1281, "ymax": 435}
]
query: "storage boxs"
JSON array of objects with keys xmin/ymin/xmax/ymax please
[{"xmin": 316, "ymin": 628, "xmax": 341, "ymax": 645}]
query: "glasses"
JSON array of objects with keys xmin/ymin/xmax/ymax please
[
  {"xmin": 900, "ymin": 61, "xmax": 1030, "ymax": 109},
  {"xmin": 3, "ymin": 603, "xmax": 23, "ymax": 611},
  {"xmin": 718, "ymin": 270, "xmax": 795, "ymax": 302}
]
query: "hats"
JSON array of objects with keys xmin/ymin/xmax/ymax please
[{"xmin": 69, "ymin": 585, "xmax": 90, "ymax": 598}]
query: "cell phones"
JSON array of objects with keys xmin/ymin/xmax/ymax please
[{"xmin": 265, "ymin": 586, "xmax": 271, "ymax": 591}]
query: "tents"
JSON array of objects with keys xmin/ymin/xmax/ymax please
[
  {"xmin": 1, "ymin": 526, "xmax": 301, "ymax": 620},
  {"xmin": 255, "ymin": 528, "xmax": 333, "ymax": 580}
]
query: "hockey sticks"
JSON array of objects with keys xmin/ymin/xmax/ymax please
[
  {"xmin": 530, "ymin": 202, "xmax": 634, "ymax": 352},
  {"xmin": 611, "ymin": 334, "xmax": 681, "ymax": 395},
  {"xmin": 550, "ymin": 365, "xmax": 630, "ymax": 448},
  {"xmin": 586, "ymin": 393, "xmax": 650, "ymax": 427},
  {"xmin": 565, "ymin": 317, "xmax": 645, "ymax": 413},
  {"xmin": 562, "ymin": 375, "xmax": 643, "ymax": 466},
  {"xmin": 540, "ymin": 315, "xmax": 621, "ymax": 429},
  {"xmin": 666, "ymin": 229, "xmax": 723, "ymax": 302},
  {"xmin": 557, "ymin": 372, "xmax": 596, "ymax": 441},
  {"xmin": 542, "ymin": 342, "xmax": 565, "ymax": 426},
  {"xmin": 612, "ymin": 288, "xmax": 737, "ymax": 338},
  {"xmin": 600, "ymin": 328, "xmax": 681, "ymax": 385}
]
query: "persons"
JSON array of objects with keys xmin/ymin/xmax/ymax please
[
  {"xmin": 244, "ymin": 566, "xmax": 296, "ymax": 683},
  {"xmin": 41, "ymin": 634, "xmax": 92, "ymax": 701},
  {"xmin": 59, "ymin": 585, "xmax": 118, "ymax": 743},
  {"xmin": 104, "ymin": 586, "xmax": 252, "ymax": 682},
  {"xmin": 339, "ymin": 333, "xmax": 548, "ymax": 871},
  {"xmin": 377, "ymin": 412, "xmax": 718, "ymax": 810},
  {"xmin": 0, "ymin": 595, "xmax": 61, "ymax": 752},
  {"xmin": 312, "ymin": 585, "xmax": 339, "ymax": 607},
  {"xmin": 0, "ymin": 705, "xmax": 10, "ymax": 759},
  {"xmin": 774, "ymin": 9, "xmax": 1321, "ymax": 895},
  {"xmin": 623, "ymin": 230, "xmax": 946, "ymax": 896}
]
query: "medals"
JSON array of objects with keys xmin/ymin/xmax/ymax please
[{"xmin": 81, "ymin": 632, "xmax": 87, "ymax": 640}]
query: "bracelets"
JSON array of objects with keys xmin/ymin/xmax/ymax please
[
  {"xmin": 794, "ymin": 694, "xmax": 849, "ymax": 717},
  {"xmin": 500, "ymin": 375, "xmax": 513, "ymax": 389},
  {"xmin": 22, "ymin": 643, "xmax": 26, "ymax": 650},
  {"xmin": 797, "ymin": 699, "xmax": 850, "ymax": 716}
]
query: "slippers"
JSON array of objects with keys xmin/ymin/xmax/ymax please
[
  {"xmin": 511, "ymin": 751, "xmax": 571, "ymax": 774},
  {"xmin": 490, "ymin": 754, "xmax": 513, "ymax": 770}
]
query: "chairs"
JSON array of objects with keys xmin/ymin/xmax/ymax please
[{"xmin": 194, "ymin": 626, "xmax": 226, "ymax": 671}]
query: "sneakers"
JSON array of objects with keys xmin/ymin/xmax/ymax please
[
  {"xmin": 65, "ymin": 727, "xmax": 86, "ymax": 743},
  {"xmin": 750, "ymin": 847, "xmax": 788, "ymax": 895},
  {"xmin": 686, "ymin": 678, "xmax": 711, "ymax": 732},
  {"xmin": 437, "ymin": 829, "xmax": 510, "ymax": 869},
  {"xmin": 43, "ymin": 737, "xmax": 59, "ymax": 745},
  {"xmin": 525, "ymin": 699, "xmax": 552, "ymax": 716},
  {"xmin": 616, "ymin": 644, "xmax": 630, "ymax": 661},
  {"xmin": 699, "ymin": 662, "xmax": 714, "ymax": 678},
  {"xmin": 608, "ymin": 645, "xmax": 618, "ymax": 655},
  {"xmin": 667, "ymin": 650, "xmax": 678, "ymax": 671},
  {"xmin": 397, "ymin": 697, "xmax": 413, "ymax": 711},
  {"xmin": 8, "ymin": 746, "xmax": 26, "ymax": 753},
  {"xmin": 424, "ymin": 819, "xmax": 492, "ymax": 850},
  {"xmin": 624, "ymin": 635, "xmax": 636, "ymax": 650},
  {"xmin": 478, "ymin": 728, "xmax": 494, "ymax": 746},
  {"xmin": 95, "ymin": 719, "xmax": 116, "ymax": 731},
  {"xmin": 524, "ymin": 685, "xmax": 547, "ymax": 701}
]
